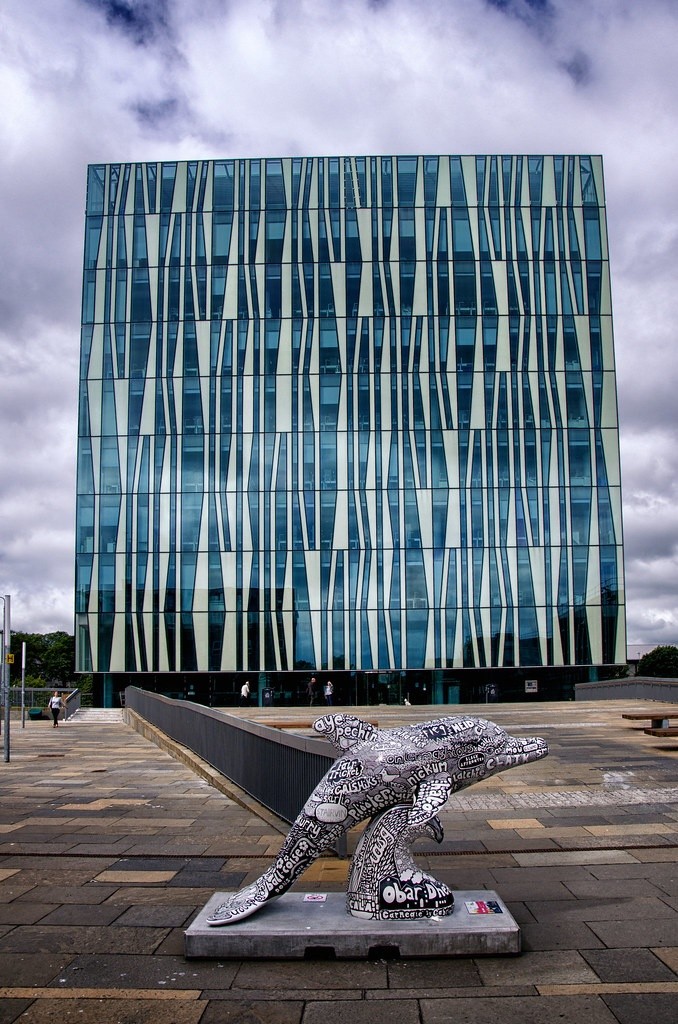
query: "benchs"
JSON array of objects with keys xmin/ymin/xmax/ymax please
[
  {"xmin": 644, "ymin": 728, "xmax": 678, "ymax": 737},
  {"xmin": 622, "ymin": 713, "xmax": 678, "ymax": 728},
  {"xmin": 247, "ymin": 719, "xmax": 378, "ymax": 739}
]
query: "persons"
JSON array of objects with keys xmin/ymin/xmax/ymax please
[
  {"xmin": 306, "ymin": 678, "xmax": 317, "ymax": 707},
  {"xmin": 324, "ymin": 680, "xmax": 334, "ymax": 706},
  {"xmin": 47, "ymin": 691, "xmax": 69, "ymax": 728},
  {"xmin": 240, "ymin": 681, "xmax": 252, "ymax": 707}
]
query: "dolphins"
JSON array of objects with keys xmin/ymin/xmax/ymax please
[{"xmin": 207, "ymin": 714, "xmax": 550, "ymax": 927}]
